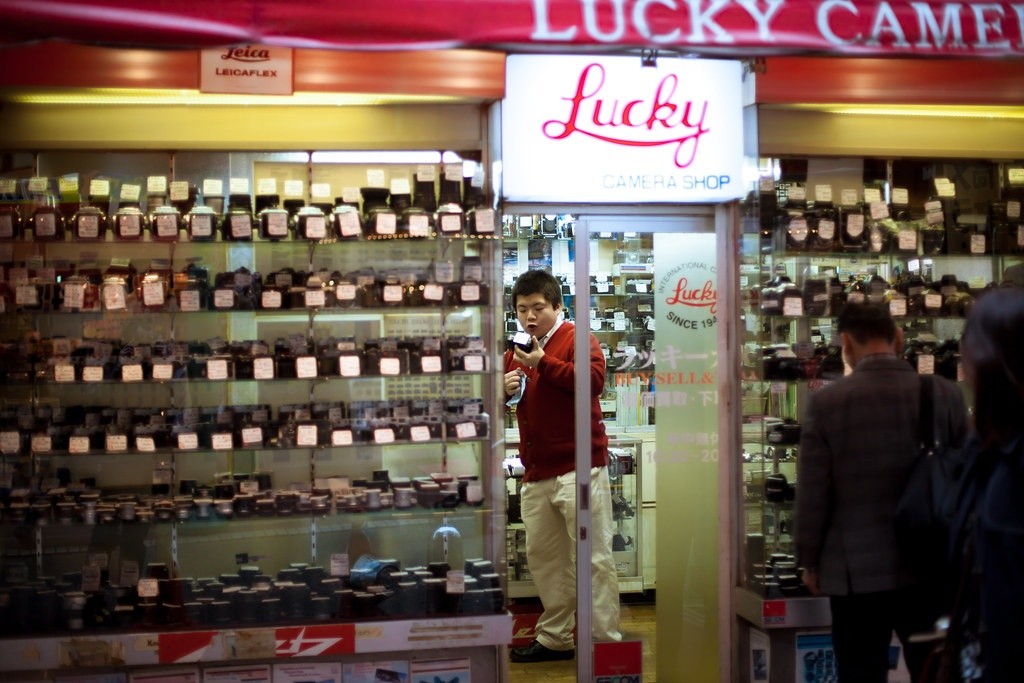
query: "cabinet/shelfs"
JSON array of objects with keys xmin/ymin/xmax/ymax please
[
  {"xmin": 0, "ymin": 93, "xmax": 507, "ymax": 682},
  {"xmin": 506, "ymin": 428, "xmax": 657, "ymax": 599},
  {"xmin": 508, "ymin": 215, "xmax": 652, "ymax": 425},
  {"xmin": 651, "ymin": 101, "xmax": 1024, "ymax": 682}
]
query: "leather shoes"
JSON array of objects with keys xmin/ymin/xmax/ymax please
[{"xmin": 510, "ymin": 641, "xmax": 575, "ymax": 663}]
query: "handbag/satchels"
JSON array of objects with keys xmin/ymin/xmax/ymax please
[{"xmin": 895, "ymin": 376, "xmax": 978, "ymax": 585}]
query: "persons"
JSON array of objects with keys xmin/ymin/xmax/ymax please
[
  {"xmin": 504, "ymin": 269, "xmax": 622, "ymax": 663},
  {"xmin": 794, "ymin": 279, "xmax": 1024, "ymax": 683}
]
